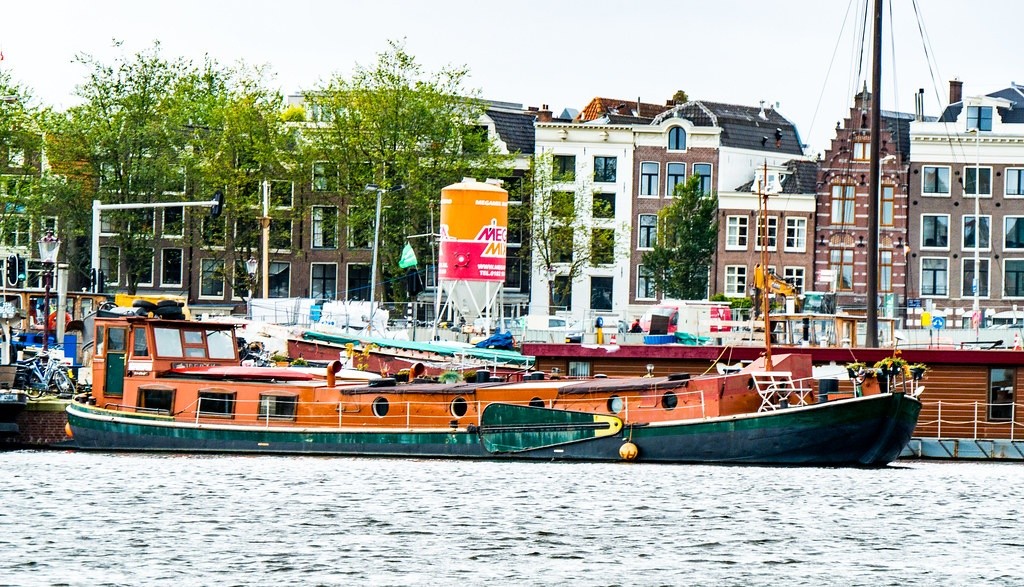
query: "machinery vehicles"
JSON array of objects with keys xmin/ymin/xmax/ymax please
[{"xmin": 749, "ymin": 261, "xmax": 839, "ymax": 342}]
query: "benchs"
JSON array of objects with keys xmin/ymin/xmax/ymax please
[{"xmin": 751, "ymin": 372, "xmax": 813, "ymax": 413}]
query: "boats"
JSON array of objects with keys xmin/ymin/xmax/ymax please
[
  {"xmin": 285, "ymin": 328, "xmax": 536, "ymax": 385},
  {"xmin": 62, "ymin": 160, "xmax": 929, "ymax": 477}
]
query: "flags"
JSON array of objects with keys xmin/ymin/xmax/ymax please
[{"xmin": 398, "ymin": 244, "xmax": 418, "ymax": 268}]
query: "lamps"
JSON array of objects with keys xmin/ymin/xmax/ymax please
[
  {"xmin": 646, "ymin": 364, "xmax": 655, "ymax": 377},
  {"xmin": 270, "ymin": 379, "xmax": 276, "ymax": 384}
]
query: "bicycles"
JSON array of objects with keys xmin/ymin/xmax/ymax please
[{"xmin": 22, "ymin": 344, "xmax": 77, "ymax": 400}]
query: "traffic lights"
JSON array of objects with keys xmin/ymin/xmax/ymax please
[
  {"xmin": 98, "ymin": 269, "xmax": 107, "ymax": 293},
  {"xmin": 90, "ymin": 268, "xmax": 98, "ymax": 285},
  {"xmin": 15, "ymin": 252, "xmax": 27, "ymax": 282},
  {"xmin": 6, "ymin": 253, "xmax": 19, "ymax": 286}
]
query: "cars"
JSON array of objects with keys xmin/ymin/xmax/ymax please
[{"xmin": 541, "ymin": 315, "xmax": 630, "ymax": 342}]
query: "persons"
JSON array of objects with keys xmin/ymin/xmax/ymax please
[{"xmin": 629, "ymin": 319, "xmax": 642, "ymax": 333}]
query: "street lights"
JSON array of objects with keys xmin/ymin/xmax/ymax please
[
  {"xmin": 966, "ymin": 127, "xmax": 982, "ymax": 336},
  {"xmin": 545, "ymin": 265, "xmax": 557, "ymax": 316},
  {"xmin": 245, "ymin": 255, "xmax": 259, "ymax": 320},
  {"xmin": 37, "ymin": 227, "xmax": 63, "ymax": 364},
  {"xmin": 360, "ymin": 181, "xmax": 406, "ymax": 331}
]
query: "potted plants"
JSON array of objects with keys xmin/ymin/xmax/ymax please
[
  {"xmin": 871, "ymin": 368, "xmax": 894, "ymax": 382},
  {"xmin": 873, "ymin": 358, "xmax": 910, "ymax": 378},
  {"xmin": 909, "ymin": 363, "xmax": 933, "ymax": 380},
  {"xmin": 847, "ymin": 360, "xmax": 867, "ymax": 378}
]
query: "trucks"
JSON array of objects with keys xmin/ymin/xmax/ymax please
[{"xmin": 639, "ymin": 304, "xmax": 732, "ymax": 333}]
